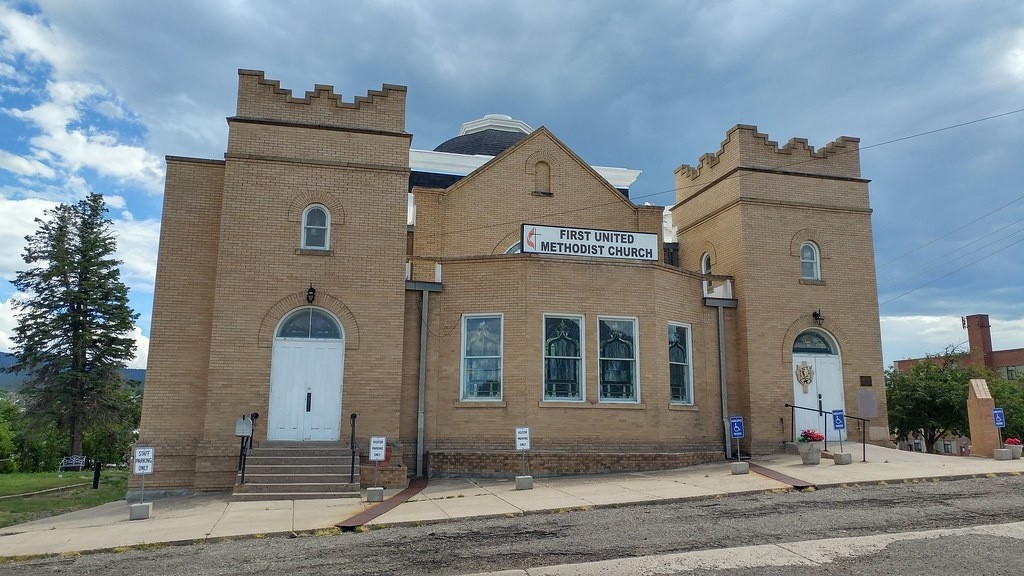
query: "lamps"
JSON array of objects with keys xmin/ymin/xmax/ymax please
[
  {"xmin": 812, "ymin": 308, "xmax": 824, "ymax": 326},
  {"xmin": 306, "ymin": 282, "xmax": 319, "ymax": 306}
]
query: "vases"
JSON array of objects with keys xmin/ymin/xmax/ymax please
[
  {"xmin": 798, "ymin": 442, "xmax": 823, "ymax": 468},
  {"xmin": 1004, "ymin": 444, "xmax": 1024, "ymax": 459}
]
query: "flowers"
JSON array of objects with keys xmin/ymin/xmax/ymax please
[
  {"xmin": 1006, "ymin": 437, "xmax": 1021, "ymax": 445},
  {"xmin": 798, "ymin": 429, "xmax": 825, "ymax": 442}
]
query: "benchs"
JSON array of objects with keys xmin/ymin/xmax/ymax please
[{"xmin": 58, "ymin": 454, "xmax": 87, "ymax": 473}]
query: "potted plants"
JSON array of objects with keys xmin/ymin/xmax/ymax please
[{"xmin": 367, "ymin": 437, "xmax": 398, "ymax": 467}]
